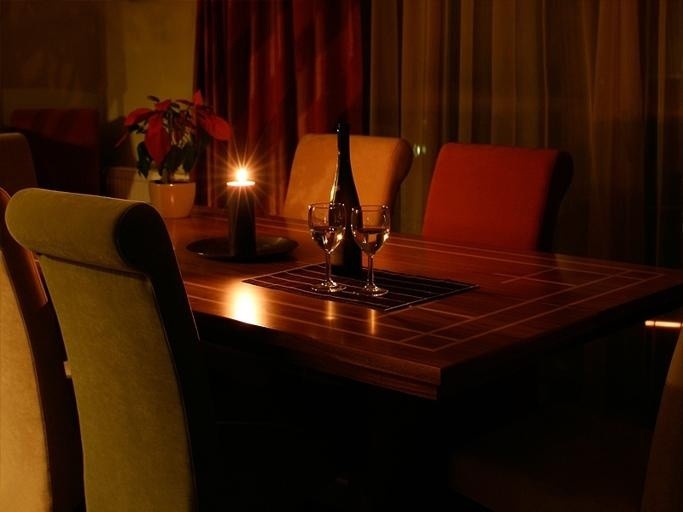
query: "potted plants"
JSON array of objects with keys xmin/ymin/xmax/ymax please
[{"xmin": 113, "ymin": 93, "xmax": 234, "ymax": 219}]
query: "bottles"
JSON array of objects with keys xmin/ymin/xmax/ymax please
[{"xmin": 328, "ymin": 120, "xmax": 364, "ymax": 276}]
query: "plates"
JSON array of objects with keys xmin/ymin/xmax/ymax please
[{"xmin": 185, "ymin": 234, "xmax": 299, "ymax": 261}]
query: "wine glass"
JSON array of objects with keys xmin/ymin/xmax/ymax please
[
  {"xmin": 307, "ymin": 202, "xmax": 346, "ymax": 294},
  {"xmin": 350, "ymin": 204, "xmax": 390, "ymax": 298}
]
